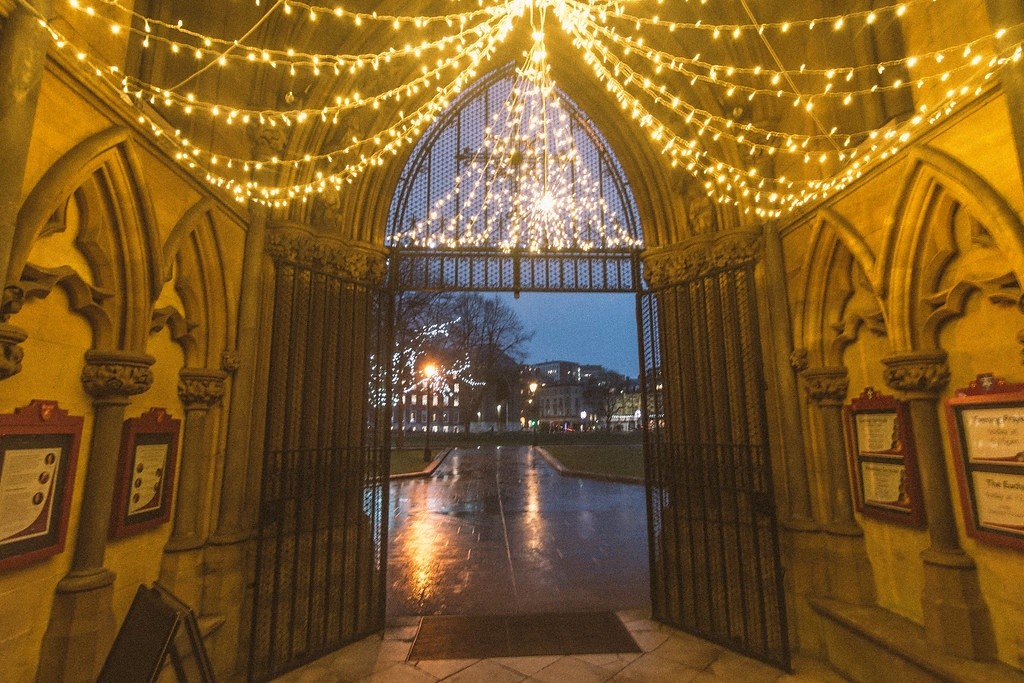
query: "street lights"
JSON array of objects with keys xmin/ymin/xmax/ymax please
[
  {"xmin": 529, "ymin": 382, "xmax": 537, "ymax": 434},
  {"xmin": 424, "ymin": 363, "xmax": 437, "ymax": 460}
]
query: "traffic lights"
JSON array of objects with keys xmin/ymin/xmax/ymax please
[{"xmin": 532, "ymin": 418, "xmax": 536, "ymax": 426}]
